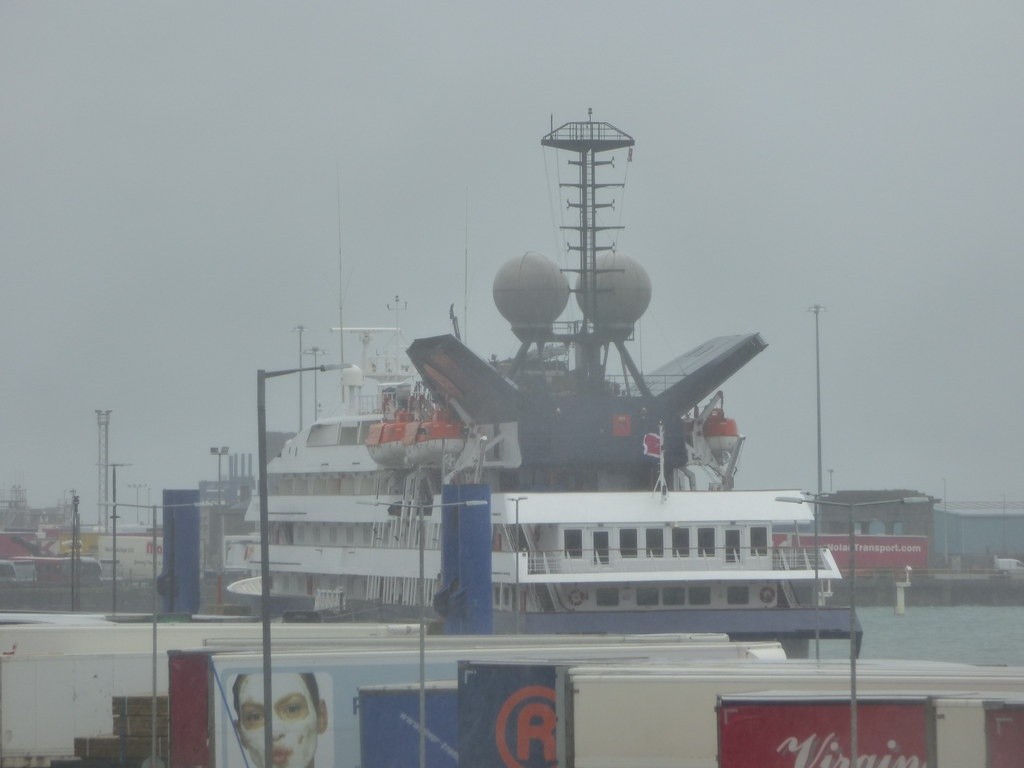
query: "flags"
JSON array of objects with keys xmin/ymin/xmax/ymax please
[{"xmin": 642, "ymin": 432, "xmax": 661, "ymax": 458}]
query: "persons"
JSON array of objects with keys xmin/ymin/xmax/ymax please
[{"xmin": 232, "ymin": 673, "xmax": 327, "ymax": 768}]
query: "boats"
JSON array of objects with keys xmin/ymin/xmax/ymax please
[
  {"xmin": 223, "ymin": 106, "xmax": 865, "ymax": 661},
  {"xmin": 365, "ymin": 422, "xmax": 464, "ymax": 470}
]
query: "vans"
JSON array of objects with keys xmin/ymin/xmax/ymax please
[{"xmin": 0, "ymin": 558, "xmax": 40, "ymax": 588}]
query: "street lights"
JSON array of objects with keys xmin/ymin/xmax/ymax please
[
  {"xmin": 255, "ymin": 362, "xmax": 353, "ymax": 768},
  {"xmin": 773, "ymin": 496, "xmax": 929, "ymax": 768},
  {"xmin": 355, "ymin": 500, "xmax": 490, "ymax": 768},
  {"xmin": 507, "ymin": 497, "xmax": 529, "ymax": 635},
  {"xmin": 99, "ymin": 501, "xmax": 215, "ymax": 767}
]
